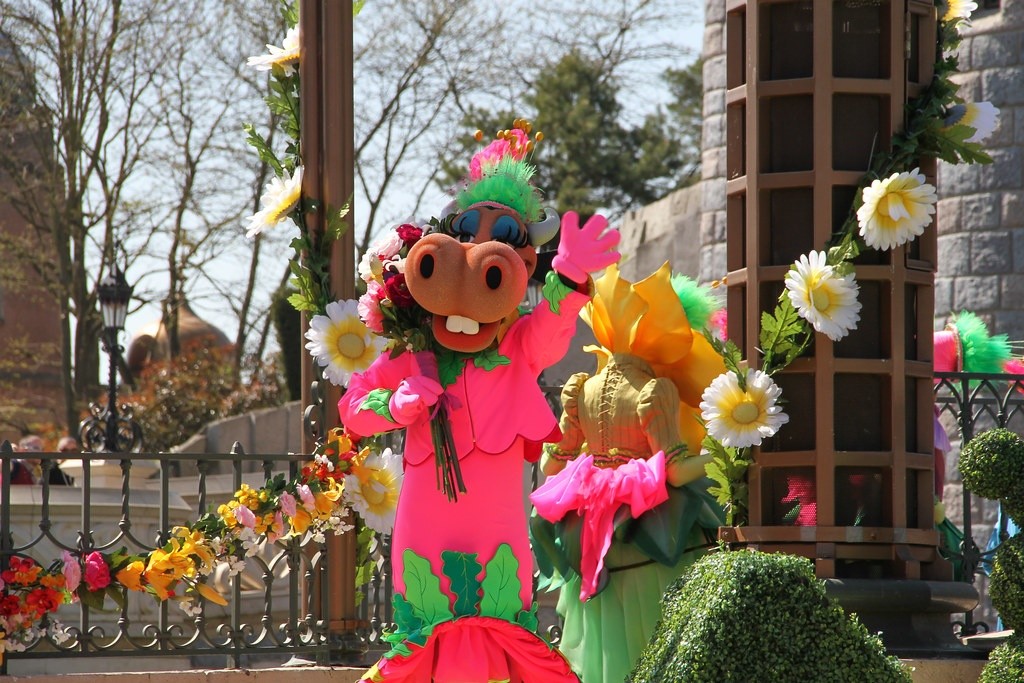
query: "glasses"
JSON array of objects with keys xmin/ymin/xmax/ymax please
[
  {"xmin": 58, "ymin": 449, "xmax": 80, "ymax": 454},
  {"xmin": 22, "ymin": 445, "xmax": 44, "ymax": 451}
]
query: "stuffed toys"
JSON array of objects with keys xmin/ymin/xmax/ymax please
[{"xmin": 337, "ymin": 118, "xmax": 621, "ymax": 683}]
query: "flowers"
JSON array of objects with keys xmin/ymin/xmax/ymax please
[
  {"xmin": 698, "ymin": 0, "xmax": 999, "ymax": 527},
  {"xmin": 0, "ymin": 428, "xmax": 368, "ymax": 666},
  {"xmin": 244, "ymin": 0, "xmax": 403, "ymax": 536},
  {"xmin": 358, "ymin": 219, "xmax": 467, "ymax": 502}
]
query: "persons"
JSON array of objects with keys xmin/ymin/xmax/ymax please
[
  {"xmin": 540, "ymin": 268, "xmax": 723, "ymax": 683},
  {"xmin": 0, "ymin": 435, "xmax": 81, "ymax": 486}
]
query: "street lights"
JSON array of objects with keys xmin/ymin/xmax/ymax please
[{"xmin": 93, "ymin": 250, "xmax": 133, "ymax": 454}]
